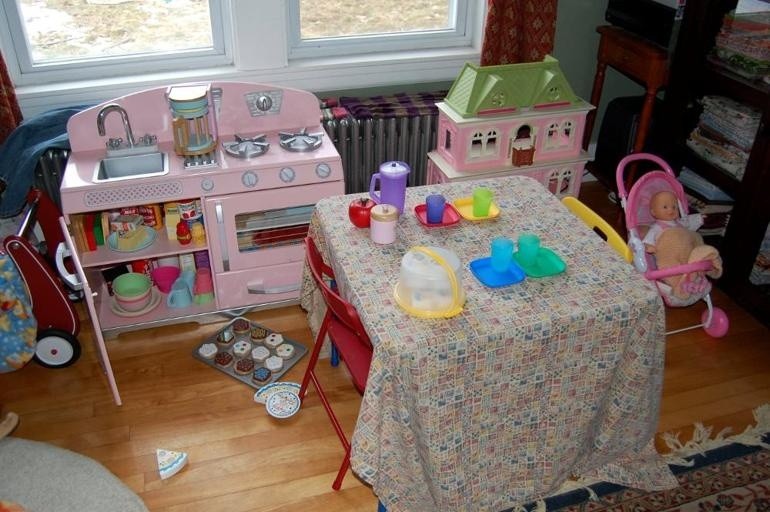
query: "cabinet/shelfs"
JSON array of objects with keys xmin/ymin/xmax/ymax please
[
  {"xmin": 657, "ymin": 65, "xmax": 769, "ymax": 331},
  {"xmin": 59, "ymin": 200, "xmax": 219, "ymax": 406}
]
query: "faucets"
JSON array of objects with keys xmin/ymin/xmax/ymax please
[{"xmin": 95, "ymin": 104, "xmax": 138, "ymax": 150}]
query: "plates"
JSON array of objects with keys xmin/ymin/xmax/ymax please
[
  {"xmin": 452, "ymin": 197, "xmax": 500, "ymax": 221},
  {"xmin": 513, "ymin": 247, "xmax": 567, "ymax": 278},
  {"xmin": 106, "ymin": 225, "xmax": 158, "ymax": 253},
  {"xmin": 268, "ymin": 391, "xmax": 301, "ymax": 419},
  {"xmin": 109, "ymin": 289, "xmax": 162, "ymax": 317},
  {"xmin": 469, "ymin": 257, "xmax": 525, "ymax": 289},
  {"xmin": 413, "ymin": 203, "xmax": 462, "ymax": 227}
]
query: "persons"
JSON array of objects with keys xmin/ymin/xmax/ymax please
[{"xmin": 642, "ymin": 191, "xmax": 706, "ymax": 256}]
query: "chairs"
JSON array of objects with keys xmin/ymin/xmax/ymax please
[
  {"xmin": 559, "ymin": 195, "xmax": 634, "ymax": 264},
  {"xmin": 297, "ymin": 236, "xmax": 374, "ymax": 493}
]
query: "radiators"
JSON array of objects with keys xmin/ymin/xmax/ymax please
[{"xmin": 35, "ymin": 92, "xmax": 437, "ymax": 194}]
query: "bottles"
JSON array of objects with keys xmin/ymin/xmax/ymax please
[{"xmin": 369, "ymin": 202, "xmax": 398, "ymax": 246}]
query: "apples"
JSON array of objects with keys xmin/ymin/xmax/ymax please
[{"xmin": 348, "ymin": 197, "xmax": 377, "ymax": 228}]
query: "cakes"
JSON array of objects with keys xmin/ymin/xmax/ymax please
[
  {"xmin": 157, "ymin": 449, "xmax": 188, "ymax": 480},
  {"xmin": 199, "ymin": 319, "xmax": 295, "ymax": 386}
]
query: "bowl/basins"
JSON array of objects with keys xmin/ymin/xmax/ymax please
[{"xmin": 112, "ymin": 273, "xmax": 151, "ymax": 313}]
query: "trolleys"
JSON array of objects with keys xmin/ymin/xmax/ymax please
[{"xmin": 1, "ymin": 185, "xmax": 88, "ymax": 376}]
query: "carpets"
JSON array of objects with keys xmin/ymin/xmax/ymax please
[
  {"xmin": 1, "ymin": 435, "xmax": 147, "ymax": 512},
  {"xmin": 496, "ymin": 403, "xmax": 769, "ymax": 512}
]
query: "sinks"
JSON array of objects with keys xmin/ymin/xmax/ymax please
[{"xmin": 92, "ymin": 151, "xmax": 168, "ymax": 182}]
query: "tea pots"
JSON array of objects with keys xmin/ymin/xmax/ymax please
[{"xmin": 367, "ymin": 159, "xmax": 413, "ymax": 219}]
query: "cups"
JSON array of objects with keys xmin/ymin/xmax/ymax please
[
  {"xmin": 153, "ymin": 265, "xmax": 214, "ymax": 310},
  {"xmin": 517, "ymin": 233, "xmax": 542, "ymax": 270},
  {"xmin": 426, "ymin": 194, "xmax": 446, "ymax": 223},
  {"xmin": 472, "ymin": 189, "xmax": 492, "ymax": 218},
  {"xmin": 490, "ymin": 237, "xmax": 515, "ymax": 273}
]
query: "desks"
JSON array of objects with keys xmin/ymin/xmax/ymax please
[
  {"xmin": 296, "ymin": 176, "xmax": 679, "ymax": 511},
  {"xmin": 581, "ymin": 26, "xmax": 669, "ymax": 199}
]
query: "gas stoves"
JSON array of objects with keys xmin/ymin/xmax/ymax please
[{"xmin": 218, "ymin": 124, "xmax": 344, "ymax": 196}]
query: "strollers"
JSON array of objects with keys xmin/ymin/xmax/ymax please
[{"xmin": 613, "ymin": 151, "xmax": 732, "ymax": 344}]
